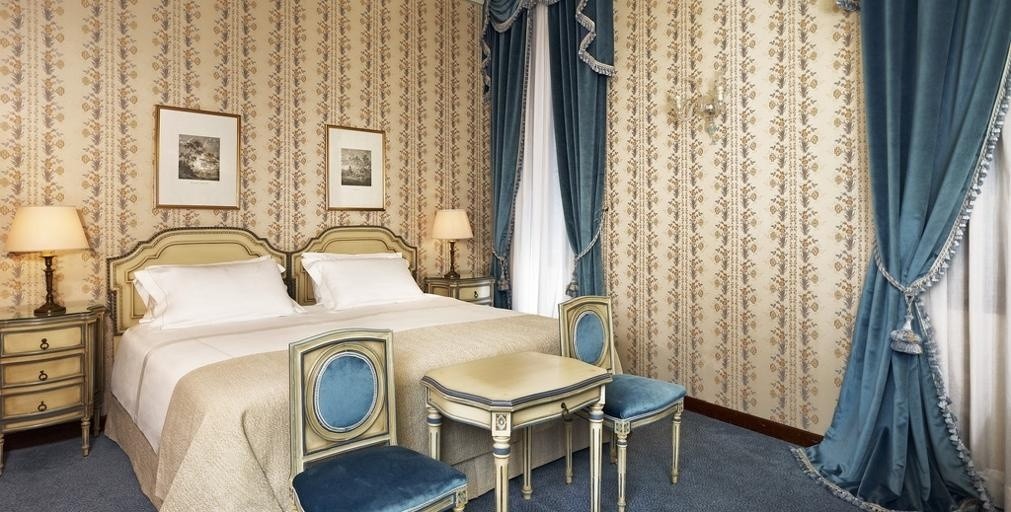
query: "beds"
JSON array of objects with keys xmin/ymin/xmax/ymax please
[{"xmin": 87, "ymin": 223, "xmax": 624, "ymax": 512}]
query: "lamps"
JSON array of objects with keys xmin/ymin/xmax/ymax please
[
  {"xmin": 3, "ymin": 203, "xmax": 92, "ymax": 317},
  {"xmin": 430, "ymin": 207, "xmax": 475, "ymax": 280}
]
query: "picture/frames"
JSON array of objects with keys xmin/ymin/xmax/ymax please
[
  {"xmin": 323, "ymin": 123, "xmax": 390, "ymax": 215},
  {"xmin": 152, "ymin": 102, "xmax": 244, "ymax": 213}
]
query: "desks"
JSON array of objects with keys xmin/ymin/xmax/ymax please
[{"xmin": 415, "ymin": 348, "xmax": 616, "ymax": 512}]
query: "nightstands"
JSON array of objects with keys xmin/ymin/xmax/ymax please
[
  {"xmin": 423, "ymin": 270, "xmax": 496, "ymax": 307},
  {"xmin": 1, "ymin": 300, "xmax": 113, "ymax": 477}
]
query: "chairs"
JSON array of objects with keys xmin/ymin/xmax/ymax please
[
  {"xmin": 556, "ymin": 293, "xmax": 688, "ymax": 512},
  {"xmin": 284, "ymin": 325, "xmax": 473, "ymax": 512}
]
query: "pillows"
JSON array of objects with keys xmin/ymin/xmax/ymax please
[
  {"xmin": 300, "ymin": 251, "xmax": 424, "ymax": 311},
  {"xmin": 130, "ymin": 253, "xmax": 304, "ymax": 330}
]
